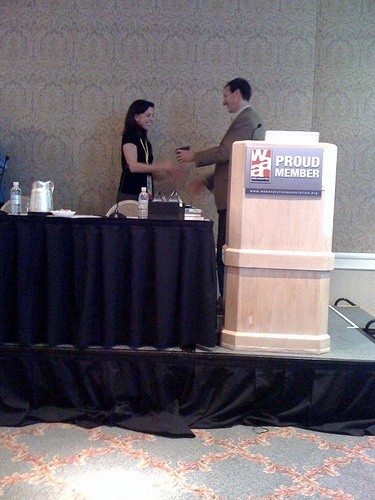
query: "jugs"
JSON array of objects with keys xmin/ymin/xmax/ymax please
[{"xmin": 29, "ymin": 180, "xmax": 54, "ymax": 215}]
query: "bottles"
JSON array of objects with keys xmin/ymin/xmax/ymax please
[
  {"xmin": 138, "ymin": 187, "xmax": 149, "ymax": 220},
  {"xmin": 9, "ymin": 181, "xmax": 22, "ymax": 215}
]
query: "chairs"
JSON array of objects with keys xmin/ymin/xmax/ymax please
[{"xmin": 105, "ymin": 200, "xmax": 139, "ymax": 217}]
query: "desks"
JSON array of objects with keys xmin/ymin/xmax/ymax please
[{"xmin": 0, "ymin": 213, "xmax": 218, "ymax": 348}]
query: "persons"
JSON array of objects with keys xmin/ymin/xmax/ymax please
[
  {"xmin": 116, "ymin": 99, "xmax": 173, "ymax": 202},
  {"xmin": 175, "ymin": 77, "xmax": 263, "ymax": 315}
]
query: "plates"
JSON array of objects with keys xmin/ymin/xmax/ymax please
[{"xmin": 51, "ymin": 210, "xmax": 76, "ymax": 217}]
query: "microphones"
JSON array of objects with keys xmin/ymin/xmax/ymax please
[
  {"xmin": 0, "ymin": 155, "xmax": 9, "ymax": 185},
  {"xmin": 109, "ymin": 163, "xmax": 129, "ymax": 219},
  {"xmin": 251, "ymin": 123, "xmax": 262, "ymax": 140}
]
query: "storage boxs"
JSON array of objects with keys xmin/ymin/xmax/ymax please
[{"xmin": 148, "ymin": 200, "xmax": 185, "ymax": 221}]
